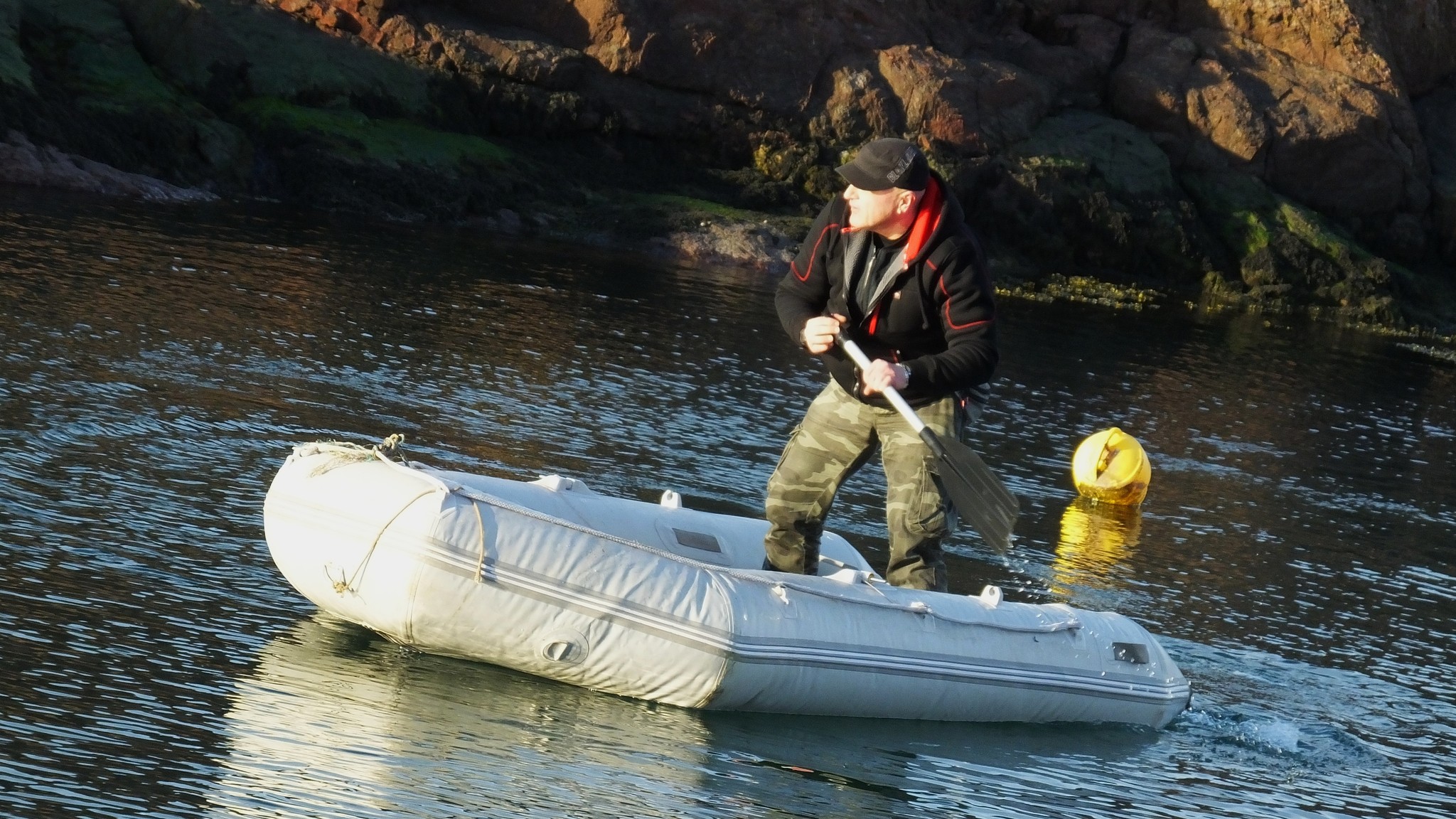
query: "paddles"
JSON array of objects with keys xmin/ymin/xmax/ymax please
[{"xmin": 819, "ymin": 309, "xmax": 1021, "ymax": 553}]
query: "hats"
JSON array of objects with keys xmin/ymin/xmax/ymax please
[{"xmin": 835, "ymin": 138, "xmax": 928, "ymax": 192}]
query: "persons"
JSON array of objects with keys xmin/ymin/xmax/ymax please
[{"xmin": 760, "ymin": 137, "xmax": 999, "ymax": 593}]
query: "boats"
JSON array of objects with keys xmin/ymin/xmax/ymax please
[{"xmin": 263, "ymin": 441, "xmax": 1193, "ymax": 730}]
